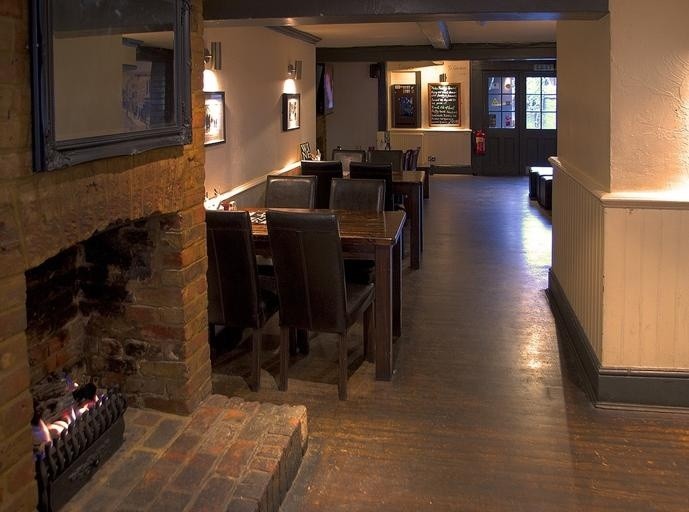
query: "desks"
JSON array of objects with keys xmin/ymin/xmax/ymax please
[{"xmin": 206, "ymin": 204, "xmax": 413, "ymax": 382}]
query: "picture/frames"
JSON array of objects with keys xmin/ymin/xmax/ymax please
[
  {"xmin": 27, "ymin": 2, "xmax": 195, "ymax": 176},
  {"xmin": 203, "ymin": 91, "xmax": 227, "ymax": 147},
  {"xmin": 280, "ymin": 93, "xmax": 301, "ymax": 131}
]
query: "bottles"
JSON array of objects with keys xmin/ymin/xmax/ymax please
[
  {"xmin": 219, "ymin": 203, "xmax": 224, "ymax": 212},
  {"xmin": 505, "ymin": 112, "xmax": 511, "ymax": 126},
  {"xmin": 316, "ymin": 148, "xmax": 322, "ymax": 161},
  {"xmin": 229, "ymin": 201, "xmax": 237, "ymax": 210},
  {"xmin": 307, "ymin": 151, "xmax": 311, "ymax": 160}
]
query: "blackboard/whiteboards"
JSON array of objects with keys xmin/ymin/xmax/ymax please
[{"xmin": 428, "ymin": 82, "xmax": 462, "ymax": 127}]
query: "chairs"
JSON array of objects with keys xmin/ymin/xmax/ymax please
[
  {"xmin": 264, "ymin": 174, "xmax": 317, "ymax": 210},
  {"xmin": 265, "ymin": 208, "xmax": 377, "ymax": 402},
  {"xmin": 205, "ymin": 206, "xmax": 311, "ymax": 393},
  {"xmin": 299, "ymin": 143, "xmax": 433, "ymax": 271}
]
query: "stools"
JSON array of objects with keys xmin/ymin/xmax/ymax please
[
  {"xmin": 527, "ymin": 165, "xmax": 554, "ymax": 200},
  {"xmin": 537, "ymin": 175, "xmax": 553, "ymax": 211}
]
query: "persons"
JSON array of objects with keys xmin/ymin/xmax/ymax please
[
  {"xmin": 289, "ymin": 101, "xmax": 297, "ymax": 122},
  {"xmin": 504, "ymin": 112, "xmax": 511, "ymax": 128},
  {"xmin": 204, "ymin": 106, "xmax": 213, "ymax": 133}
]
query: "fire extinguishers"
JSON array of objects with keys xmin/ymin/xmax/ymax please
[{"xmin": 472, "ymin": 128, "xmax": 486, "ymax": 156}]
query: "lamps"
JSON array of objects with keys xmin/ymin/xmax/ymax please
[
  {"xmin": 287, "ymin": 58, "xmax": 302, "ymax": 83},
  {"xmin": 204, "ymin": 41, "xmax": 224, "ymax": 73}
]
